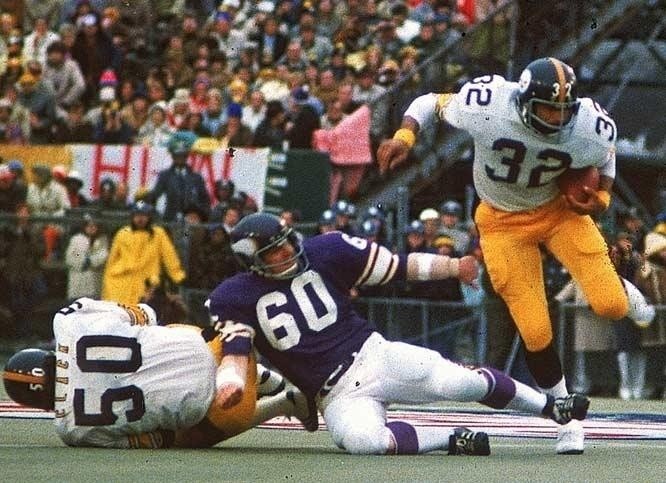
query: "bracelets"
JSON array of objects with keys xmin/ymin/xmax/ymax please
[
  {"xmin": 390, "ymin": 128, "xmax": 417, "ymax": 150},
  {"xmin": 597, "ymin": 191, "xmax": 613, "ymax": 209}
]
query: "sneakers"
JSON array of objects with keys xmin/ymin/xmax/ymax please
[
  {"xmin": 256, "ymin": 364, "xmax": 285, "ymax": 396},
  {"xmin": 289, "ymin": 385, "xmax": 320, "ymax": 432},
  {"xmin": 555, "ymin": 425, "xmax": 585, "ymax": 454},
  {"xmin": 621, "ymin": 276, "xmax": 653, "ymax": 328},
  {"xmin": 449, "ymin": 427, "xmax": 492, "ymax": 456},
  {"xmin": 552, "ymin": 396, "xmax": 590, "ymax": 424}
]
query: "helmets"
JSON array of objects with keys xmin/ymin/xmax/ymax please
[
  {"xmin": 3, "ymin": 347, "xmax": 54, "ymax": 411},
  {"xmin": 517, "ymin": 56, "xmax": 580, "ymax": 144},
  {"xmin": 1, "ymin": 144, "xmax": 234, "ymax": 214},
  {"xmin": 319, "ymin": 201, "xmax": 465, "ymax": 247},
  {"xmin": 230, "ymin": 214, "xmax": 310, "ymax": 280}
]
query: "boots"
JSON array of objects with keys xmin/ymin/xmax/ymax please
[{"xmin": 617, "ymin": 351, "xmax": 647, "ymax": 400}]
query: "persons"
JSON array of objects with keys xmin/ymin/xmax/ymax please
[
  {"xmin": 372, "ymin": 49, "xmax": 658, "ymax": 455},
  {"xmin": 1, "ymin": 294, "xmax": 322, "ymax": 449},
  {"xmin": 199, "ymin": 207, "xmax": 593, "ymax": 457}
]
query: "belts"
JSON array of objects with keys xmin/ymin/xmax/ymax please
[{"xmin": 322, "ymin": 345, "xmax": 359, "ymax": 398}]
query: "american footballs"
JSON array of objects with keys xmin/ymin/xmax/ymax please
[{"xmin": 561, "ymin": 168, "xmax": 600, "ymax": 204}]
